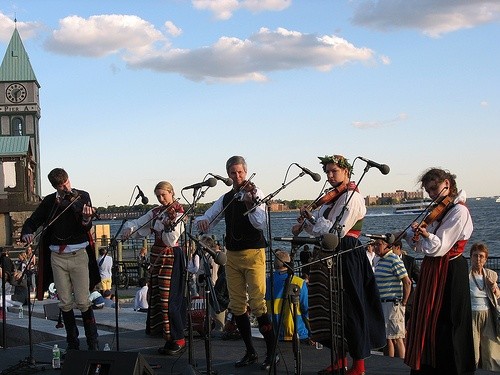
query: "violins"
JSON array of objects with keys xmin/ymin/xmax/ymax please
[
  {"xmin": 234, "ymin": 181, "xmax": 261, "ymax": 213},
  {"xmin": 55, "ymin": 188, "xmax": 101, "ymax": 221},
  {"xmin": 412, "ymin": 196, "xmax": 453, "ymax": 243},
  {"xmin": 152, "ymin": 203, "xmax": 177, "ymax": 232},
  {"xmin": 297, "ymin": 181, "xmax": 346, "ymax": 222}
]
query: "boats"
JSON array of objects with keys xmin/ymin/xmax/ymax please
[{"xmin": 393, "ymin": 196, "xmax": 437, "ymax": 215}]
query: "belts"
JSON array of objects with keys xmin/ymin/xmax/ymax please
[{"xmin": 382, "ymin": 299, "xmax": 394, "ymax": 303}]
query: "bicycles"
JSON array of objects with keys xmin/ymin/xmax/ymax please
[{"xmin": 112, "ymin": 264, "xmax": 130, "ymax": 290}]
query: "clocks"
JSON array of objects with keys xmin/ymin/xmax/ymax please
[{"xmin": 6, "ymin": 83, "xmax": 27, "ymax": 102}]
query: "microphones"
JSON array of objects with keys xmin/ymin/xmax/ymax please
[
  {"xmin": 184, "ymin": 178, "xmax": 217, "ymax": 189},
  {"xmin": 137, "ymin": 186, "xmax": 148, "ymax": 204},
  {"xmin": 273, "ymin": 232, "xmax": 339, "ymax": 251},
  {"xmin": 361, "ymin": 233, "xmax": 396, "ymax": 245},
  {"xmin": 295, "ymin": 163, "xmax": 321, "ymax": 182},
  {"xmin": 64, "ymin": 190, "xmax": 78, "ymax": 197},
  {"xmin": 208, "ymin": 173, "xmax": 234, "ymax": 186},
  {"xmin": 358, "ymin": 156, "xmax": 390, "ymax": 175},
  {"xmin": 187, "ymin": 233, "xmax": 227, "ymax": 266}
]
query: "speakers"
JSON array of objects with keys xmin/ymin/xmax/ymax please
[{"xmin": 61, "ymin": 349, "xmax": 157, "ymax": 375}]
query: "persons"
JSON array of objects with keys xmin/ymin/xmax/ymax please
[
  {"xmin": 364, "ymin": 238, "xmax": 420, "ymax": 359},
  {"xmin": 264, "ymin": 251, "xmax": 311, "ymax": 340},
  {"xmin": 179, "ymin": 235, "xmax": 231, "ymax": 333},
  {"xmin": 392, "ymin": 168, "xmax": 477, "ymax": 375},
  {"xmin": 291, "ymin": 155, "xmax": 386, "ymax": 374},
  {"xmin": 88, "ymin": 247, "xmax": 150, "ymax": 312},
  {"xmin": 0, "ymin": 246, "xmax": 59, "ymax": 299},
  {"xmin": 21, "ymin": 168, "xmax": 102, "ymax": 353},
  {"xmin": 467, "ymin": 243, "xmax": 500, "ymax": 372},
  {"xmin": 122, "ymin": 181, "xmax": 189, "ymax": 356},
  {"xmin": 300, "ymin": 244, "xmax": 313, "ymax": 276},
  {"xmin": 194, "ymin": 156, "xmax": 280, "ymax": 371}
]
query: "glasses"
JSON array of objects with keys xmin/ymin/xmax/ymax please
[{"xmin": 473, "ymin": 254, "xmax": 484, "ymax": 259}]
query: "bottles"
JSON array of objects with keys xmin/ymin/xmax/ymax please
[
  {"xmin": 104, "ymin": 343, "xmax": 110, "ymax": 351},
  {"xmin": 316, "ymin": 342, "xmax": 323, "ymax": 349},
  {"xmin": 18, "ymin": 305, "xmax": 23, "ymax": 319},
  {"xmin": 52, "ymin": 344, "xmax": 60, "ymax": 368}
]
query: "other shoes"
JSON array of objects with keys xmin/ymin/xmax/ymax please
[
  {"xmin": 159, "ymin": 336, "xmax": 185, "ymax": 355},
  {"xmin": 235, "ymin": 352, "xmax": 258, "ymax": 366},
  {"xmin": 260, "ymin": 355, "xmax": 280, "ymax": 370},
  {"xmin": 317, "ymin": 365, "xmax": 348, "ymax": 375}
]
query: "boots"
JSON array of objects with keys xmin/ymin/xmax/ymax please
[
  {"xmin": 81, "ymin": 307, "xmax": 99, "ymax": 350},
  {"xmin": 62, "ymin": 309, "xmax": 81, "ymax": 352}
]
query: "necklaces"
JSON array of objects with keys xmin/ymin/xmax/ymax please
[{"xmin": 472, "ymin": 269, "xmax": 485, "ymax": 291}]
